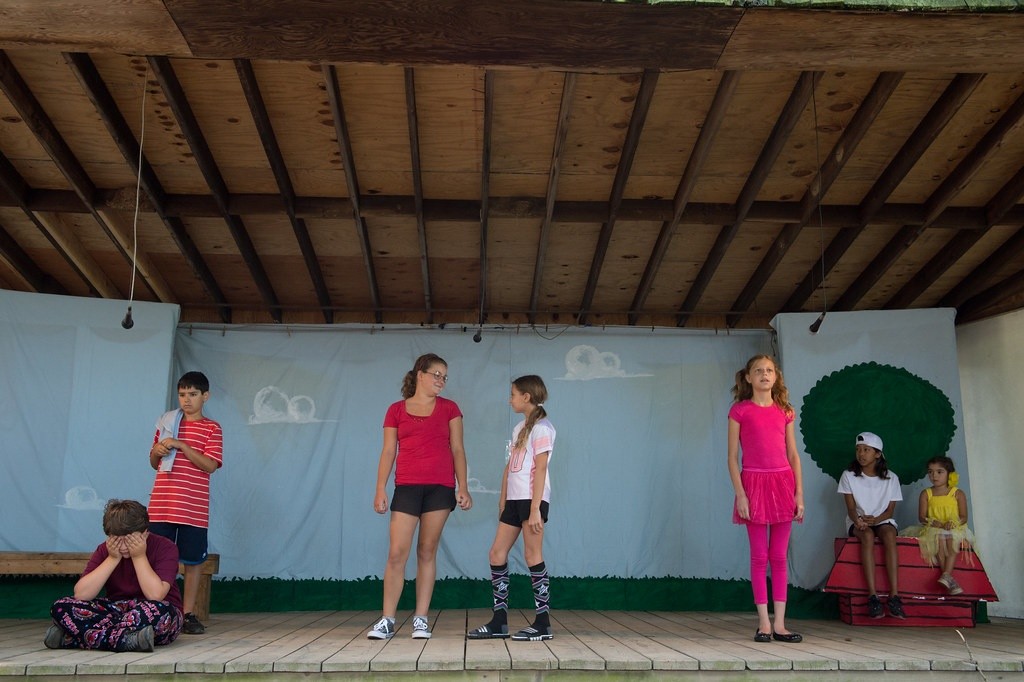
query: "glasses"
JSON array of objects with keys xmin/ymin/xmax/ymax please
[{"xmin": 422, "ymin": 370, "xmax": 449, "ymax": 383}]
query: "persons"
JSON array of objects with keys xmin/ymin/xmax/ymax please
[
  {"xmin": 728, "ymin": 353, "xmax": 804, "ymax": 644},
  {"xmin": 367, "ymin": 353, "xmax": 473, "ymax": 639},
  {"xmin": 897, "ymin": 456, "xmax": 980, "ymax": 595},
  {"xmin": 147, "ymin": 370, "xmax": 222, "ymax": 634},
  {"xmin": 837, "ymin": 432, "xmax": 906, "ymax": 619},
  {"xmin": 467, "ymin": 375, "xmax": 556, "ymax": 641},
  {"xmin": 44, "ymin": 499, "xmax": 184, "ymax": 653}
]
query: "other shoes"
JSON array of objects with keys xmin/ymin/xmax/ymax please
[
  {"xmin": 43, "ymin": 623, "xmax": 66, "ymax": 649},
  {"xmin": 948, "ymin": 582, "xmax": 964, "ymax": 596},
  {"xmin": 119, "ymin": 625, "xmax": 154, "ymax": 652},
  {"xmin": 867, "ymin": 595, "xmax": 885, "ymax": 619},
  {"xmin": 937, "ymin": 571, "xmax": 953, "ymax": 589},
  {"xmin": 885, "ymin": 595, "xmax": 905, "ymax": 619},
  {"xmin": 754, "ymin": 627, "xmax": 771, "ymax": 642},
  {"xmin": 773, "ymin": 631, "xmax": 803, "ymax": 643},
  {"xmin": 183, "ymin": 612, "xmax": 204, "ymax": 634}
]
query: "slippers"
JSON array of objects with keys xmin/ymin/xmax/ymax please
[
  {"xmin": 467, "ymin": 622, "xmax": 510, "ymax": 638},
  {"xmin": 511, "ymin": 624, "xmax": 554, "ymax": 641}
]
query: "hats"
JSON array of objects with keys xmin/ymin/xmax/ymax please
[{"xmin": 856, "ymin": 432, "xmax": 886, "ymax": 460}]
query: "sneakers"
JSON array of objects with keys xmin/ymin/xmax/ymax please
[
  {"xmin": 411, "ymin": 616, "xmax": 432, "ymax": 639},
  {"xmin": 367, "ymin": 618, "xmax": 396, "ymax": 640}
]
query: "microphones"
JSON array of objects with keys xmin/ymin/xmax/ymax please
[
  {"xmin": 121, "ymin": 307, "xmax": 134, "ymax": 329},
  {"xmin": 808, "ymin": 312, "xmax": 826, "ymax": 334},
  {"xmin": 473, "ymin": 327, "xmax": 483, "ymax": 342}
]
